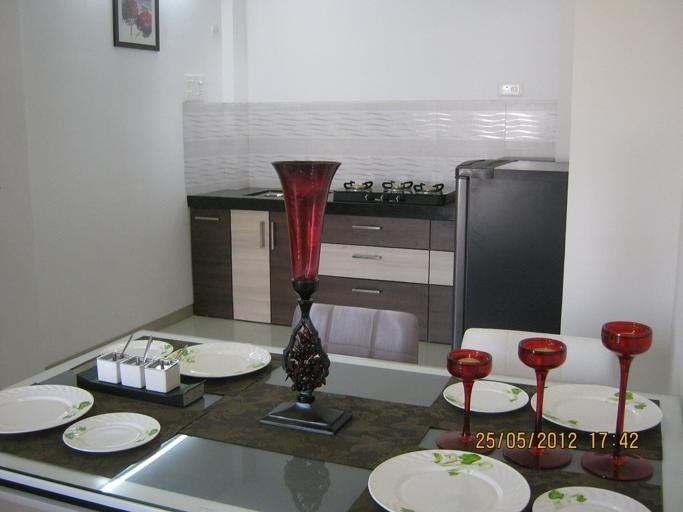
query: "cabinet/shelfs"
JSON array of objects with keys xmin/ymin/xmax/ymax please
[
  {"xmin": 188, "ymin": 209, "xmax": 233, "ymax": 320},
  {"xmin": 312, "ymin": 214, "xmax": 430, "ymax": 342},
  {"xmin": 230, "ymin": 210, "xmax": 298, "ymax": 327}
]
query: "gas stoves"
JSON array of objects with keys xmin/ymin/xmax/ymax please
[{"xmin": 336, "ymin": 179, "xmax": 457, "ymax": 207}]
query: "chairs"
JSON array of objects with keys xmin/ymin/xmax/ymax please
[
  {"xmin": 460, "ymin": 329, "xmax": 621, "ymax": 387},
  {"xmin": 290, "ymin": 304, "xmax": 419, "ymax": 365}
]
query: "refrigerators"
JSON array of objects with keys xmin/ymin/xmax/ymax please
[{"xmin": 454, "ymin": 156, "xmax": 568, "ymax": 349}]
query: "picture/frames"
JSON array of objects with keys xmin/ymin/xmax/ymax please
[{"xmin": 112, "ymin": 0, "xmax": 160, "ymax": 51}]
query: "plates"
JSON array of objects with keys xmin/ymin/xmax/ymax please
[
  {"xmin": 368, "ymin": 450, "xmax": 654, "ymax": 512},
  {"xmin": 442, "ymin": 378, "xmax": 663, "ymax": 434},
  {"xmin": 103, "ymin": 337, "xmax": 273, "ymax": 377},
  {"xmin": 2, "ymin": 383, "xmax": 162, "ymax": 453}
]
